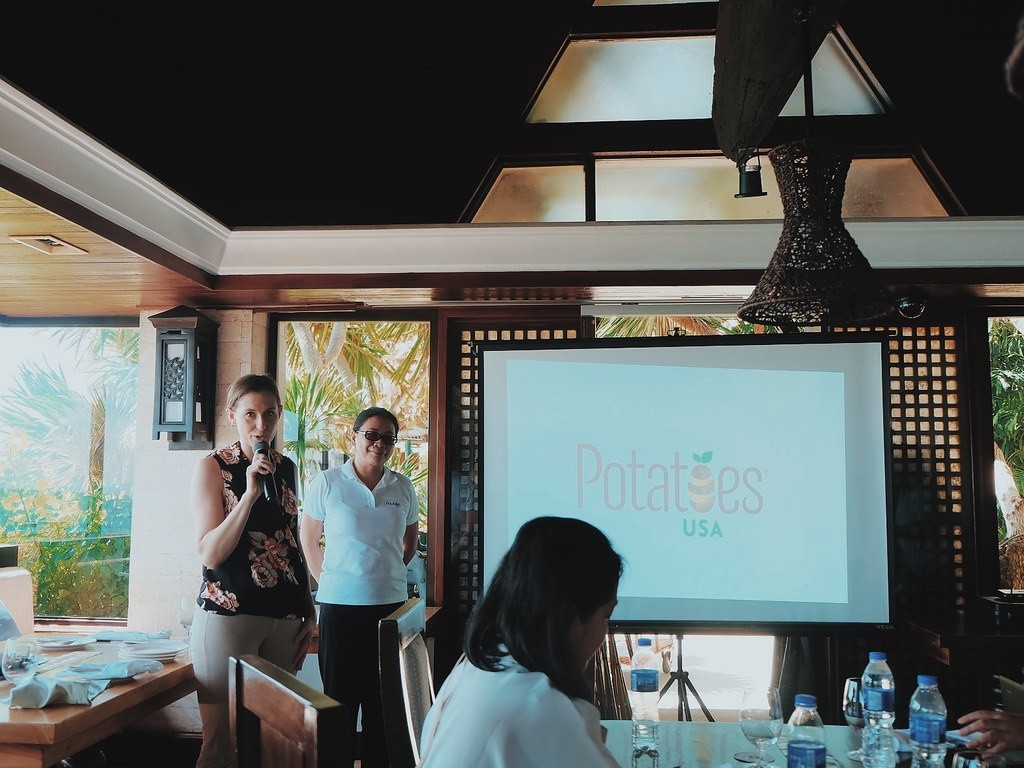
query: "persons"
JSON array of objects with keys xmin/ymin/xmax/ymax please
[
  {"xmin": 187, "ymin": 374, "xmax": 317, "ymax": 768},
  {"xmin": 958, "ymin": 710, "xmax": 1024, "ymax": 760},
  {"xmin": 419, "ymin": 517, "xmax": 624, "ymax": 768},
  {"xmin": 300, "ymin": 407, "xmax": 420, "ymax": 768}
]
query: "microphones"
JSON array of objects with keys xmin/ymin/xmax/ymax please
[{"xmin": 253, "ymin": 440, "xmax": 276, "ymax": 502}]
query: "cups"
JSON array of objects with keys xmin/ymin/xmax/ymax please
[
  {"xmin": 1, "ymin": 637, "xmax": 39, "ymax": 686},
  {"xmin": 951, "ymin": 751, "xmax": 1006, "ymax": 768}
]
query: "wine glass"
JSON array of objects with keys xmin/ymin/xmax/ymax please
[
  {"xmin": 842, "ymin": 676, "xmax": 865, "ymax": 762},
  {"xmin": 739, "ymin": 686, "xmax": 784, "ymax": 768}
]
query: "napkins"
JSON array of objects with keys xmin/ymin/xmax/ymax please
[
  {"xmin": 57, "ymin": 659, "xmax": 165, "ymax": 679},
  {"xmin": 89, "ymin": 630, "xmax": 172, "ymax": 640},
  {"xmin": 945, "ymin": 729, "xmax": 1024, "ymax": 761},
  {"xmin": 9, "ymin": 677, "xmax": 111, "ymax": 708}
]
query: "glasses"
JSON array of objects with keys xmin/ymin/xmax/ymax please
[{"xmin": 355, "ymin": 431, "xmax": 398, "ymax": 446}]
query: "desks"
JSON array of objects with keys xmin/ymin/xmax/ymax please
[
  {"xmin": 600, "ymin": 720, "xmax": 968, "ymax": 768},
  {"xmin": 0, "ymin": 633, "xmax": 198, "ymax": 768}
]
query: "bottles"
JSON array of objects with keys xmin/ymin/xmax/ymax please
[
  {"xmin": 631, "ymin": 638, "xmax": 660, "ymax": 752},
  {"xmin": 786, "ymin": 694, "xmax": 828, "ymax": 768},
  {"xmin": 860, "ymin": 652, "xmax": 897, "ymax": 768},
  {"xmin": 909, "ymin": 675, "xmax": 947, "ymax": 768}
]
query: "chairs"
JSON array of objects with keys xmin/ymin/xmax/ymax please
[
  {"xmin": 227, "ymin": 654, "xmax": 353, "ymax": 767},
  {"xmin": 377, "ymin": 598, "xmax": 436, "ymax": 768}
]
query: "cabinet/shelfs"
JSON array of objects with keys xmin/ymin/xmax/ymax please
[{"xmin": 904, "ymin": 615, "xmax": 1024, "ymax": 729}]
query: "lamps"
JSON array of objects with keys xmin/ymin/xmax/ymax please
[
  {"xmin": 147, "ymin": 305, "xmax": 221, "ymax": 442},
  {"xmin": 739, "ymin": 5, "xmax": 896, "ymax": 326},
  {"xmin": 735, "ymin": 146, "xmax": 767, "ymax": 199}
]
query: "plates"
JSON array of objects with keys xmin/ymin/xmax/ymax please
[
  {"xmin": 35, "ymin": 635, "xmax": 97, "ymax": 650},
  {"xmin": 118, "ymin": 641, "xmax": 189, "ymax": 663}
]
